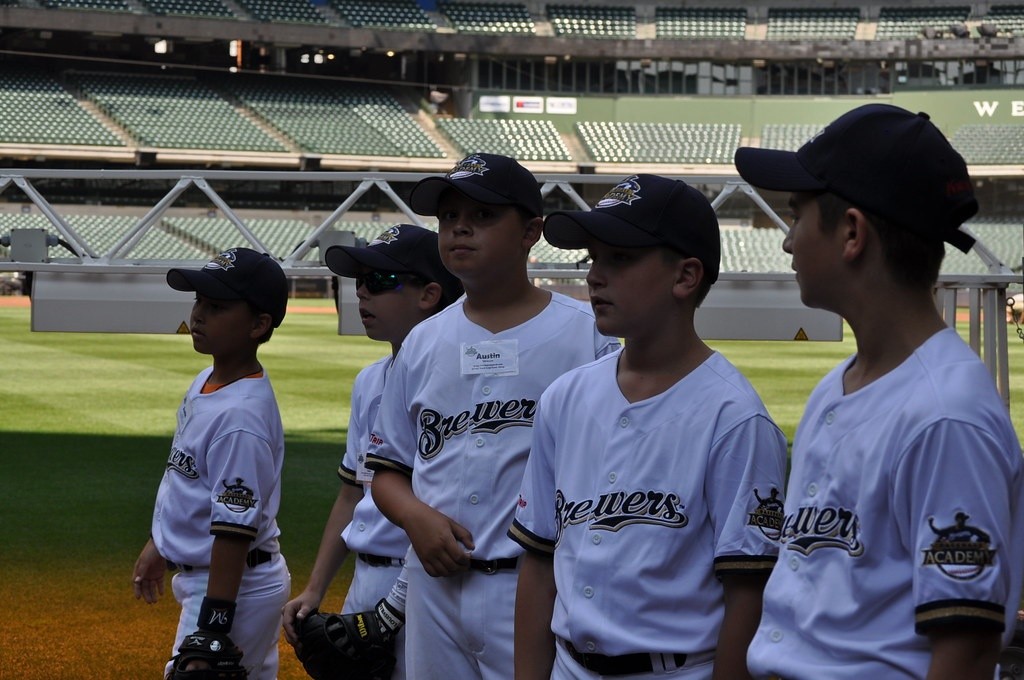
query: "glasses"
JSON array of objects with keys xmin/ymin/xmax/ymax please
[{"xmin": 355, "ymin": 270, "xmax": 427, "ymax": 296}]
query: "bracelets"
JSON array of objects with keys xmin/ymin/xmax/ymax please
[{"xmin": 197, "ymin": 596, "xmax": 238, "ymax": 633}]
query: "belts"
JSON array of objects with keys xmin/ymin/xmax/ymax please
[
  {"xmin": 165, "ymin": 547, "xmax": 271, "ymax": 571},
  {"xmin": 470, "ymin": 555, "xmax": 518, "ymax": 575},
  {"xmin": 564, "ymin": 632, "xmax": 688, "ymax": 676},
  {"xmin": 358, "ymin": 551, "xmax": 403, "ymax": 567}
]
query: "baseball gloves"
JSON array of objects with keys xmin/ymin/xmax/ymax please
[
  {"xmin": 293, "ymin": 598, "xmax": 405, "ymax": 680},
  {"xmin": 165, "ymin": 596, "xmax": 249, "ymax": 680}
]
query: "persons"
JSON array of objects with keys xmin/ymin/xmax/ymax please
[
  {"xmin": 507, "ymin": 174, "xmax": 789, "ymax": 680},
  {"xmin": 365, "ymin": 152, "xmax": 622, "ymax": 680},
  {"xmin": 132, "ymin": 248, "xmax": 289, "ymax": 680},
  {"xmin": 280, "ymin": 224, "xmax": 464, "ymax": 680},
  {"xmin": 734, "ymin": 104, "xmax": 1024, "ymax": 680}
]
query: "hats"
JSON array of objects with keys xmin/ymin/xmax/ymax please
[
  {"xmin": 409, "ymin": 153, "xmax": 543, "ymax": 233},
  {"xmin": 166, "ymin": 249, "xmax": 288, "ymax": 327},
  {"xmin": 542, "ymin": 174, "xmax": 720, "ymax": 284},
  {"xmin": 734, "ymin": 105, "xmax": 979, "ymax": 254},
  {"xmin": 325, "ymin": 224, "xmax": 465, "ymax": 300}
]
